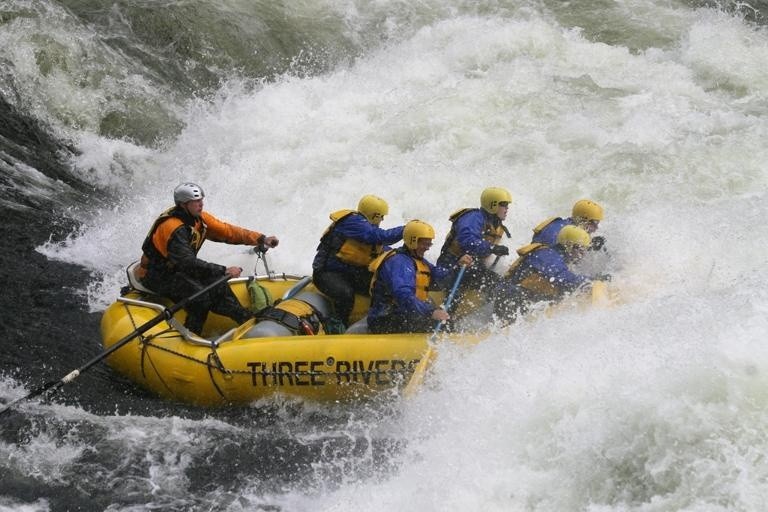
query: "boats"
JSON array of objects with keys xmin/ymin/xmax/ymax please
[{"xmin": 100, "ymin": 275, "xmax": 623, "ymax": 405}]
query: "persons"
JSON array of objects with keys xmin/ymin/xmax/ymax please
[
  {"xmin": 493, "ymin": 226, "xmax": 612, "ymax": 324},
  {"xmin": 531, "ymin": 200, "xmax": 607, "ymax": 255},
  {"xmin": 139, "ymin": 183, "xmax": 279, "ymax": 336},
  {"xmin": 368, "ymin": 220, "xmax": 473, "ymax": 334},
  {"xmin": 313, "ymin": 195, "xmax": 406, "ymax": 329},
  {"xmin": 437, "ymin": 187, "xmax": 512, "ymax": 287}
]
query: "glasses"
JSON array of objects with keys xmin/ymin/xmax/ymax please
[{"xmin": 498, "ymin": 202, "xmax": 509, "ymax": 207}]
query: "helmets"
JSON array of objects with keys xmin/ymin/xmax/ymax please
[
  {"xmin": 573, "ymin": 200, "xmax": 603, "ymax": 222},
  {"xmin": 481, "ymin": 186, "xmax": 512, "ymax": 215},
  {"xmin": 173, "ymin": 183, "xmax": 206, "ymax": 202},
  {"xmin": 557, "ymin": 225, "xmax": 590, "ymax": 249},
  {"xmin": 358, "ymin": 195, "xmax": 388, "ymax": 224},
  {"xmin": 403, "ymin": 220, "xmax": 435, "ymax": 249}
]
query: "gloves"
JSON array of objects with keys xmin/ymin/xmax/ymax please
[
  {"xmin": 491, "ymin": 244, "xmax": 508, "ymax": 256},
  {"xmin": 599, "ymin": 274, "xmax": 610, "ymax": 282},
  {"xmin": 592, "ymin": 236, "xmax": 604, "ymax": 250}
]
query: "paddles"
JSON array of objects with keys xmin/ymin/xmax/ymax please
[
  {"xmin": 400, "ymin": 252, "xmax": 472, "ymax": 408},
  {"xmin": 0, "ymin": 268, "xmax": 243, "ymax": 417}
]
